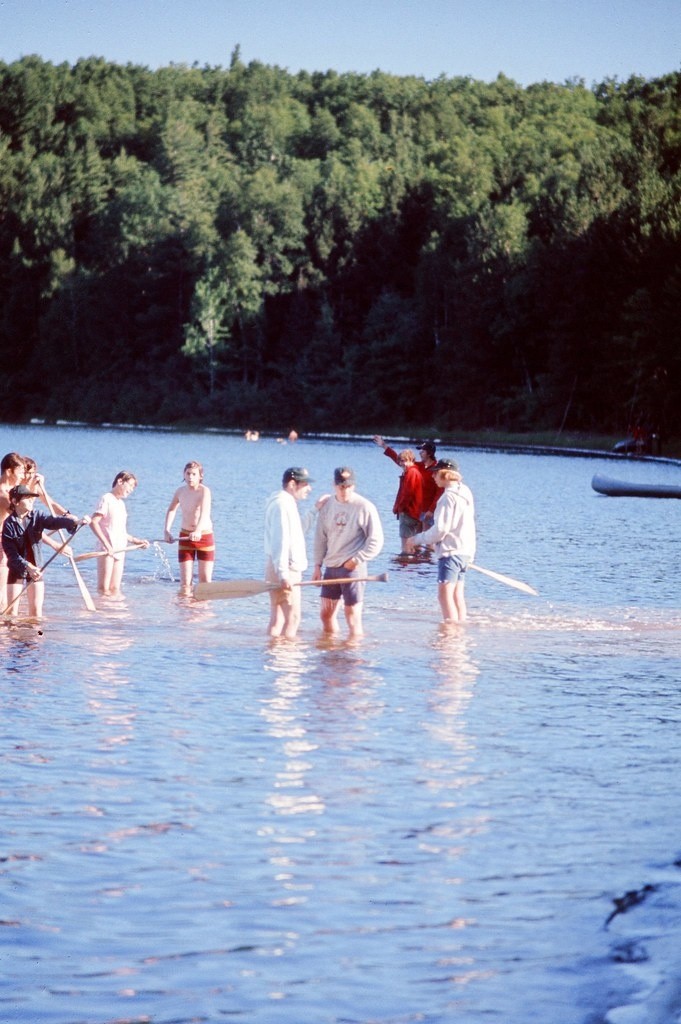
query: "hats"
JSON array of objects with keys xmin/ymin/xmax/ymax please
[
  {"xmin": 283, "ymin": 467, "xmax": 314, "ymax": 485},
  {"xmin": 416, "ymin": 441, "xmax": 436, "ymax": 452},
  {"xmin": 9, "ymin": 484, "xmax": 40, "ymax": 497},
  {"xmin": 426, "ymin": 459, "xmax": 458, "ymax": 471},
  {"xmin": 334, "ymin": 466, "xmax": 356, "ymax": 486}
]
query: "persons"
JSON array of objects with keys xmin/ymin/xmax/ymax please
[
  {"xmin": 392, "ymin": 449, "xmax": 423, "ymax": 556},
  {"xmin": 0, "ymin": 453, "xmax": 80, "ymax": 615},
  {"xmin": 1, "ymin": 484, "xmax": 94, "ymax": 619},
  {"xmin": 405, "ymin": 457, "xmax": 476, "ymax": 619},
  {"xmin": 312, "ymin": 466, "xmax": 384, "ymax": 635},
  {"xmin": 165, "ymin": 462, "xmax": 215, "ymax": 586},
  {"xmin": 88, "ymin": 469, "xmax": 150, "ymax": 593},
  {"xmin": 259, "ymin": 467, "xmax": 318, "ymax": 637},
  {"xmin": 374, "ymin": 434, "xmax": 446, "ymax": 558}
]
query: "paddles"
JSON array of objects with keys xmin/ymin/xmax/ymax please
[
  {"xmin": 2, "ymin": 522, "xmax": 86, "ymax": 615},
  {"xmin": 74, "ymin": 541, "xmax": 149, "ymax": 562},
  {"xmin": 192, "ymin": 571, "xmax": 388, "ymax": 600},
  {"xmin": 146, "ymin": 536, "xmax": 189, "ymax": 543},
  {"xmin": 413, "ymin": 543, "xmax": 538, "ymax": 596},
  {"xmin": 35, "ymin": 476, "xmax": 95, "ymax": 612},
  {"xmin": 47, "ymin": 528, "xmax": 58, "ymax": 536}
]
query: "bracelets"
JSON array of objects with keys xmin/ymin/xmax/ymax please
[{"xmin": 62, "ymin": 510, "xmax": 70, "ymax": 517}]
